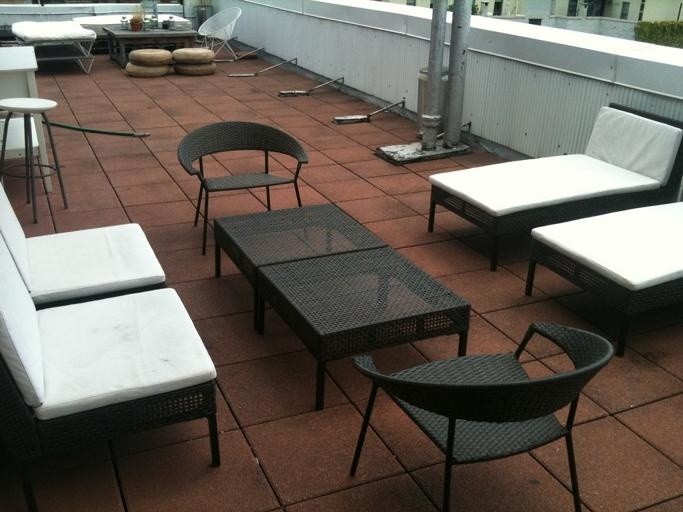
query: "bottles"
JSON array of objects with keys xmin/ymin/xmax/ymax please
[
  {"xmin": 142, "ymin": 18, "xmax": 151, "ymax": 31},
  {"xmin": 162, "ymin": 15, "xmax": 176, "ymax": 30},
  {"xmin": 120, "ymin": 16, "xmax": 128, "ymax": 30},
  {"xmin": 151, "ymin": 15, "xmax": 158, "ymax": 29}
]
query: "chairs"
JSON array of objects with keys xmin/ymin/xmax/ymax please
[
  {"xmin": 198, "ymin": 6, "xmax": 241, "ymax": 61},
  {"xmin": 526, "ymin": 202, "xmax": 682, "ymax": 336},
  {"xmin": 428, "ymin": 100, "xmax": 683, "ymax": 337},
  {"xmin": 0, "ymin": 235, "xmax": 221, "ymax": 508},
  {"xmin": 348, "ymin": 320, "xmax": 614, "ymax": 511},
  {"xmin": 429, "ymin": 102, "xmax": 683, "ymax": 273},
  {"xmin": 177, "ymin": 120, "xmax": 310, "ymax": 254},
  {"xmin": 0, "ymin": 182, "xmax": 166, "ymax": 312}
]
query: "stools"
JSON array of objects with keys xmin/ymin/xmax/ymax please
[
  {"xmin": 0, "ymin": 98, "xmax": 69, "ymax": 224},
  {"xmin": 125, "ymin": 48, "xmax": 217, "ymax": 79}
]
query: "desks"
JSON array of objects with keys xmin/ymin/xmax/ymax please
[
  {"xmin": 258, "ymin": 244, "xmax": 472, "ymax": 408},
  {"xmin": 0, "ymin": 46, "xmax": 54, "ymax": 194},
  {"xmin": 213, "ymin": 204, "xmax": 390, "ymax": 329},
  {"xmin": 9, "ymin": 18, "xmax": 97, "ymax": 73}
]
